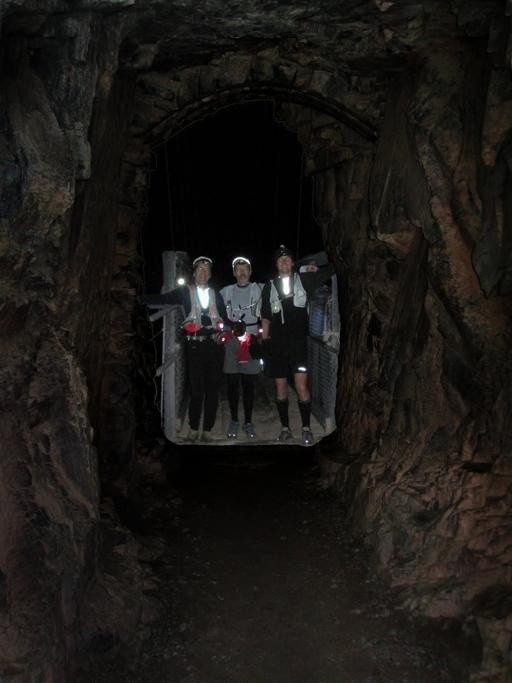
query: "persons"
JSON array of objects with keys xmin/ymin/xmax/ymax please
[
  {"xmin": 261, "ymin": 243, "xmax": 349, "ymax": 443},
  {"xmin": 140, "ymin": 255, "xmax": 246, "ymax": 441},
  {"xmin": 217, "ymin": 256, "xmax": 320, "ymax": 438}
]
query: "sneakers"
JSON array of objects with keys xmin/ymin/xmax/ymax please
[{"xmin": 185, "ymin": 426, "xmax": 314, "ymax": 443}]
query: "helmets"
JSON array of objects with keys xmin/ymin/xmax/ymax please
[
  {"xmin": 193, "ymin": 255, "xmax": 212, "ymax": 264},
  {"xmin": 232, "ymin": 256, "xmax": 251, "ymax": 268},
  {"xmin": 271, "ymin": 244, "xmax": 296, "ymax": 268}
]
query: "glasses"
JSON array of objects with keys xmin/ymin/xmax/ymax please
[
  {"xmin": 234, "ymin": 259, "xmax": 245, "ymax": 264},
  {"xmin": 194, "ymin": 260, "xmax": 210, "ymax": 265}
]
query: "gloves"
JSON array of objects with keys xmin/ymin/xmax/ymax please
[
  {"xmin": 262, "ymin": 338, "xmax": 273, "ymax": 355},
  {"xmin": 250, "ymin": 340, "xmax": 264, "ymax": 359},
  {"xmin": 231, "ymin": 321, "xmax": 246, "ymax": 335}
]
